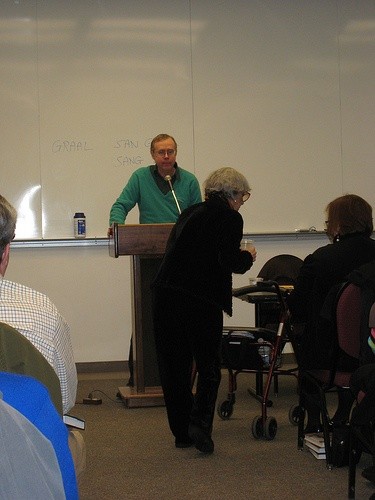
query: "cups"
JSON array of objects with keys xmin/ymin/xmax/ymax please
[
  {"xmin": 241, "ymin": 240, "xmax": 254, "ymax": 251},
  {"xmin": 248, "ymin": 278, "xmax": 263, "ymax": 285}
]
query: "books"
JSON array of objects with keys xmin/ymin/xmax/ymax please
[
  {"xmin": 304, "ymin": 434, "xmax": 332, "ymax": 460},
  {"xmin": 63, "ymin": 414, "xmax": 85, "ymax": 430}
]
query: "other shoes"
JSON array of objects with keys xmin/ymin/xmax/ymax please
[
  {"xmin": 175, "ymin": 435, "xmax": 194, "ymax": 448},
  {"xmin": 299, "ymin": 404, "xmax": 324, "ymax": 429},
  {"xmin": 361, "ymin": 465, "xmax": 375, "ymax": 482},
  {"xmin": 195, "ymin": 435, "xmax": 214, "ymax": 451}
]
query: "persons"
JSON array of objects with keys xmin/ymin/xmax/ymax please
[
  {"xmin": 108, "ymin": 133, "xmax": 204, "ymax": 399},
  {"xmin": 151, "ymin": 166, "xmax": 256, "ymax": 454},
  {"xmin": 0, "ymin": 371, "xmax": 79, "ymax": 500},
  {"xmin": 288, "ymin": 194, "xmax": 375, "ymax": 432},
  {"xmin": 0, "ymin": 195, "xmax": 86, "ymax": 479}
]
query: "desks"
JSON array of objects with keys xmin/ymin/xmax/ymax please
[{"xmin": 247, "ymin": 285, "xmax": 294, "ymax": 406}]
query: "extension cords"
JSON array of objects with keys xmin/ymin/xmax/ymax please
[{"xmin": 83, "ymin": 398, "xmax": 102, "ymax": 405}]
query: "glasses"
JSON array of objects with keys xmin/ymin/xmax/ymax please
[
  {"xmin": 240, "ymin": 190, "xmax": 250, "ymax": 202},
  {"xmin": 324, "ymin": 220, "xmax": 329, "ymax": 226},
  {"xmin": 157, "ymin": 149, "xmax": 177, "ymax": 158}
]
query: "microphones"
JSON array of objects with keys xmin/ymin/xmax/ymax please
[{"xmin": 164, "ymin": 174, "xmax": 174, "ymax": 191}]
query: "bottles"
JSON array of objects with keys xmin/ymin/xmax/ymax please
[{"xmin": 73, "ymin": 213, "xmax": 86, "ymax": 239}]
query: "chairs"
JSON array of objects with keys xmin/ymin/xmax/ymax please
[
  {"xmin": 0, "ymin": 322, "xmax": 78, "ymax": 500},
  {"xmin": 298, "ymin": 262, "xmax": 375, "ymax": 500}
]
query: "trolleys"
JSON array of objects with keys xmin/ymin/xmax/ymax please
[{"xmin": 216, "ymin": 279, "xmax": 307, "ymax": 440}]
